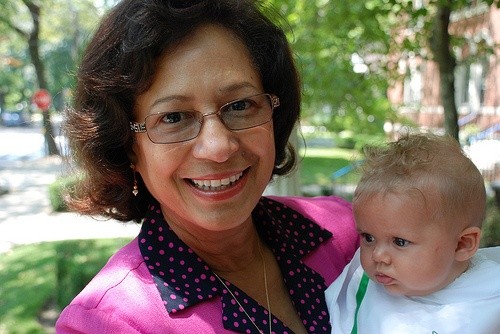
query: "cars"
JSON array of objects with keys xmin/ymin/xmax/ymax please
[{"xmin": 2, "ymin": 108, "xmax": 32, "ymax": 127}]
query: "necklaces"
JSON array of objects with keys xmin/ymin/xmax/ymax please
[{"xmin": 211, "ymin": 231, "xmax": 272, "ymax": 334}]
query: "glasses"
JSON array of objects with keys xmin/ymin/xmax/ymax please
[{"xmin": 131, "ymin": 92, "xmax": 283, "ymax": 146}]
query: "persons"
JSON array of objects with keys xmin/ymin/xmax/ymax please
[
  {"xmin": 52, "ymin": 0, "xmax": 361, "ymax": 333},
  {"xmin": 321, "ymin": 130, "xmax": 500, "ymax": 334}
]
